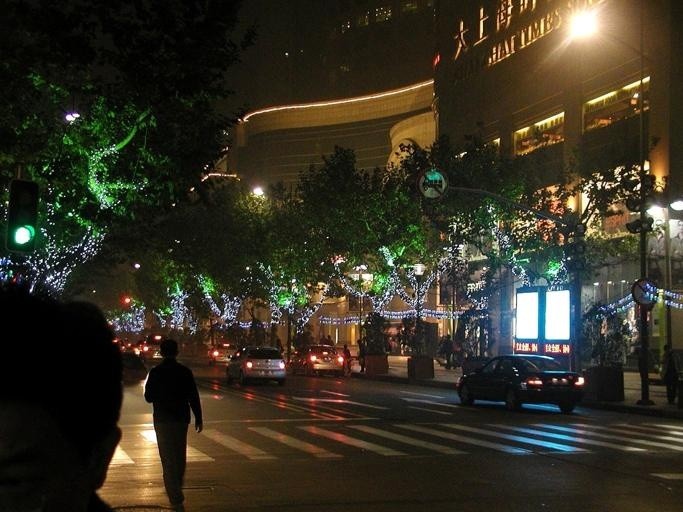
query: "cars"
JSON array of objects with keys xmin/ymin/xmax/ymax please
[
  {"xmin": 225, "ymin": 345, "xmax": 290, "ymax": 390},
  {"xmin": 455, "ymin": 353, "xmax": 588, "ymax": 420},
  {"xmin": 292, "ymin": 343, "xmax": 347, "ymax": 381},
  {"xmin": 113, "ymin": 332, "xmax": 169, "ymax": 385},
  {"xmin": 210, "ymin": 343, "xmax": 238, "ymax": 360}
]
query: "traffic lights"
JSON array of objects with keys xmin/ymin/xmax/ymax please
[
  {"xmin": 622, "ymin": 177, "xmax": 643, "ymax": 236},
  {"xmin": 7, "ymin": 179, "xmax": 41, "ymax": 258},
  {"xmin": 568, "ymin": 222, "xmax": 590, "ymax": 271},
  {"xmin": 642, "ymin": 172, "xmax": 657, "ymax": 235}
]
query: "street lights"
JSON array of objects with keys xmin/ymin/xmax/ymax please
[
  {"xmin": 650, "ymin": 171, "xmax": 683, "ymax": 356},
  {"xmin": 411, "ymin": 261, "xmax": 427, "ymax": 359},
  {"xmin": 568, "ymin": 1, "xmax": 660, "ymax": 408},
  {"xmin": 352, "ymin": 262, "xmax": 371, "ymax": 366}
]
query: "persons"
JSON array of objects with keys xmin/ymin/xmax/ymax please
[
  {"xmin": 0, "ymin": 281, "xmax": 126, "ymax": 512},
  {"xmin": 320, "ymin": 334, "xmax": 328, "ymax": 345},
  {"xmin": 607, "ymin": 332, "xmax": 627, "ymax": 368},
  {"xmin": 326, "ymin": 335, "xmax": 334, "ymax": 346},
  {"xmin": 659, "ymin": 343, "xmax": 683, "ymax": 405},
  {"xmin": 357, "ymin": 339, "xmax": 367, "ymax": 372},
  {"xmin": 343, "ymin": 344, "xmax": 354, "ymax": 374},
  {"xmin": 441, "ymin": 334, "xmax": 451, "ymax": 370},
  {"xmin": 451, "ymin": 335, "xmax": 460, "ymax": 370},
  {"xmin": 147, "ymin": 338, "xmax": 204, "ymax": 512}
]
